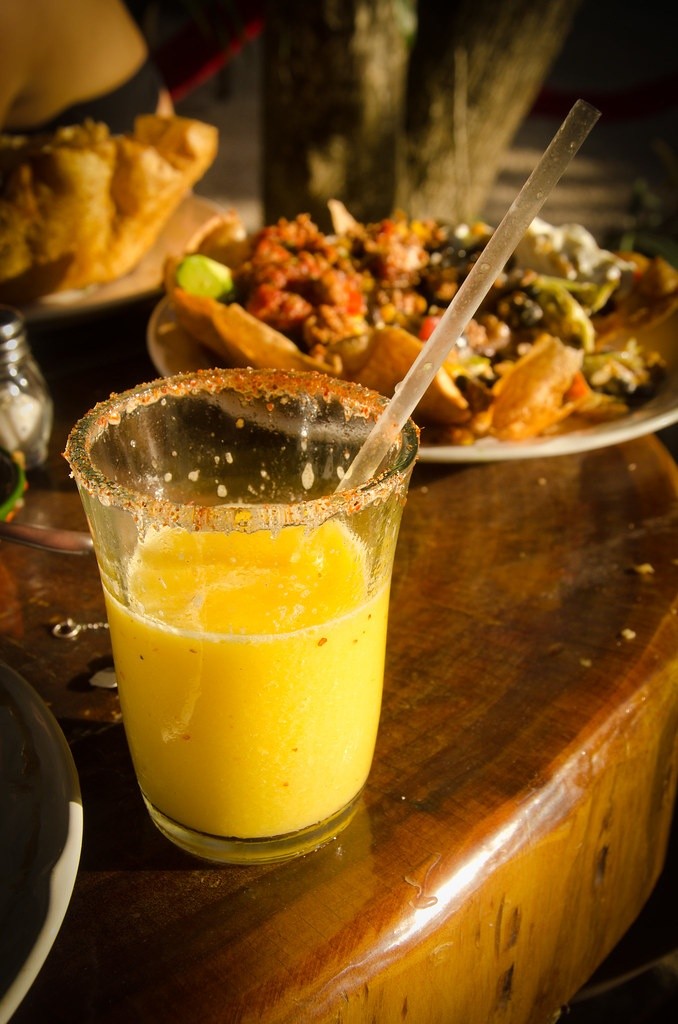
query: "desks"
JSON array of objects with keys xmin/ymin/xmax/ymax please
[{"xmin": 0, "ymin": 292, "xmax": 678, "ymax": 1024}]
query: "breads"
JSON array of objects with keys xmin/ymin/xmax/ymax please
[{"xmin": 0, "ymin": 0, "xmax": 221, "ymax": 292}]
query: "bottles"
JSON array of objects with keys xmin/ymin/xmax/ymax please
[{"xmin": 1, "ymin": 306, "xmax": 55, "ymax": 472}]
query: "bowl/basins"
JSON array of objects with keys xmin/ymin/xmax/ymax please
[{"xmin": 0, "ymin": 659, "xmax": 85, "ymax": 1024}]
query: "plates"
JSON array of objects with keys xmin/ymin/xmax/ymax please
[
  {"xmin": 20, "ymin": 195, "xmax": 225, "ymax": 322},
  {"xmin": 146, "ymin": 295, "xmax": 677, "ymax": 459}
]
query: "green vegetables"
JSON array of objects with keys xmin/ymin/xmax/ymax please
[{"xmin": 406, "ymin": 215, "xmax": 672, "ymax": 407}]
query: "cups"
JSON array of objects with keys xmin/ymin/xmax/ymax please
[{"xmin": 63, "ymin": 366, "xmax": 420, "ymax": 865}]
